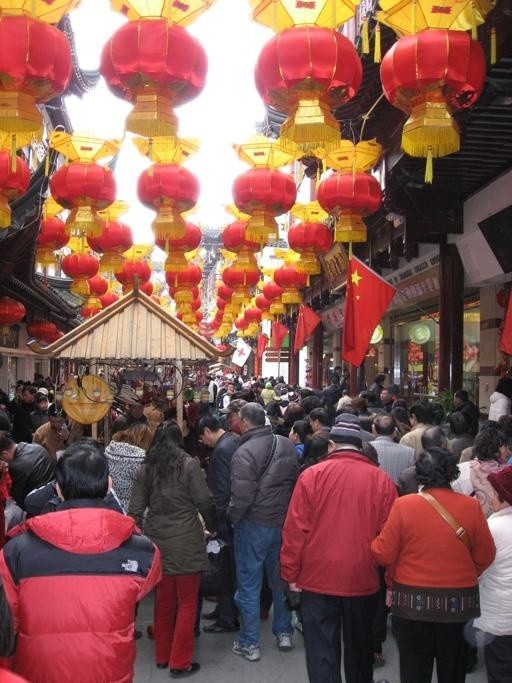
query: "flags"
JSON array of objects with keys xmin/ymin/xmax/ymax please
[
  {"xmin": 340, "ymin": 253, "xmax": 395, "ymax": 368},
  {"xmin": 257, "ymin": 334, "xmax": 271, "ymax": 357},
  {"xmin": 272, "ymin": 321, "xmax": 295, "ymax": 352},
  {"xmin": 293, "ymin": 305, "xmax": 341, "ymax": 353},
  {"xmin": 230, "ymin": 336, "xmax": 252, "ymax": 369}
]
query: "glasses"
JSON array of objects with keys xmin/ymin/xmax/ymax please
[{"xmin": 197, "ymin": 430, "xmax": 205, "ymax": 443}]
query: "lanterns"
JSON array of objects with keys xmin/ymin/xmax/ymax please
[
  {"xmin": 99, "ymin": 1, "xmax": 216, "ymax": 135},
  {"xmin": 317, "ymin": 139, "xmax": 384, "ymax": 241},
  {"xmin": 165, "ymin": 260, "xmax": 202, "ymax": 315},
  {"xmin": 82, "ymin": 271, "xmax": 109, "ymax": 311},
  {"xmin": 121, "ymin": 279, "xmax": 153, "ymax": 296},
  {"xmin": 274, "ymin": 249, "xmax": 310, "ymax": 317},
  {"xmin": 35, "ymin": 215, "xmax": 69, "ymax": 273},
  {"xmin": 114, "ymin": 244, "xmax": 155, "ymax": 282},
  {"xmin": 223, "ymin": 203, "xmax": 268, "ymax": 286},
  {"xmin": 233, "ymin": 142, "xmax": 305, "ymax": 257},
  {"xmin": 0, "ymin": 128, "xmax": 44, "ymax": 228},
  {"xmin": 0, "ymin": 296, "xmax": 26, "ymax": 333},
  {"xmin": 362, "ymin": 1, "xmax": 492, "ymax": 182},
  {"xmin": 155, "ymin": 222, "xmax": 201, "ymax": 287},
  {"xmin": 137, "ymin": 136, "xmax": 202, "ymax": 253},
  {"xmin": 80, "ymin": 303, "xmax": 102, "ymax": 317},
  {"xmin": 251, "ymin": 294, "xmax": 275, "ymax": 326},
  {"xmin": 61, "ymin": 235, "xmax": 99, "ymax": 303},
  {"xmin": 27, "ymin": 321, "xmax": 57, "ymax": 341},
  {"xmin": 0, "ymin": 1, "xmax": 81, "ymax": 169},
  {"xmin": 243, "ymin": 307, "xmax": 263, "ymax": 335},
  {"xmin": 216, "ymin": 282, "xmax": 235, "ymax": 298},
  {"xmin": 86, "ymin": 199, "xmax": 132, "ymax": 289},
  {"xmin": 151, "ymin": 298, "xmax": 241, "ymax": 336},
  {"xmin": 48, "ymin": 134, "xmax": 121, "ymax": 237},
  {"xmin": 100, "ymin": 293, "xmax": 118, "ymax": 306},
  {"xmin": 249, "ymin": 2, "xmax": 364, "ymax": 157},
  {"xmin": 288, "ymin": 200, "xmax": 334, "ymax": 287},
  {"xmin": 261, "ymin": 267, "xmax": 286, "ymax": 317}
]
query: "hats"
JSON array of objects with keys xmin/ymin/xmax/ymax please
[
  {"xmin": 487, "ymin": 465, "xmax": 512, "ymax": 507},
  {"xmin": 288, "ymin": 391, "xmax": 298, "ymax": 401},
  {"xmin": 329, "ymin": 421, "xmax": 363, "ymax": 447},
  {"xmin": 37, "ymin": 387, "xmax": 48, "ymax": 395}
]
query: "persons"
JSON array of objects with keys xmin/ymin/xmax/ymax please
[
  {"xmin": 29, "ymin": 370, "xmax": 65, "ymax": 430},
  {"xmin": 226, "ymin": 402, "xmax": 298, "ymax": 661},
  {"xmin": 396, "ymin": 427, "xmax": 460, "ymax": 495},
  {"xmin": 23, "ymin": 478, "xmax": 125, "ymax": 513},
  {"xmin": 471, "ymin": 463, "xmax": 512, "ymax": 683},
  {"xmin": 0, "ymin": 372, "xmax": 37, "ymax": 442},
  {"xmin": 185, "ymin": 365, "xmax": 214, "ymax": 467},
  {"xmin": 129, "ymin": 420, "xmax": 220, "ymax": 676},
  {"xmin": 106, "ymin": 372, "xmax": 174, "ymax": 446},
  {"xmin": 370, "ymin": 375, "xmax": 512, "ymax": 460},
  {"xmin": 204, "ymin": 365, "xmax": 307, "ymax": 438},
  {"xmin": 32, "ymin": 403, "xmax": 85, "ymax": 457},
  {"xmin": 286, "ymin": 367, "xmax": 375, "ymax": 460},
  {"xmin": 103, "ymin": 425, "xmax": 154, "ymax": 515},
  {"xmin": 0, "ymin": 442, "xmax": 163, "ymax": 683},
  {"xmin": 195, "ymin": 412, "xmax": 243, "ymax": 633},
  {"xmin": 366, "ymin": 416, "xmax": 414, "ymax": 486},
  {"xmin": 279, "ymin": 420, "xmax": 400, "ymax": 682},
  {"xmin": 375, "ymin": 369, "xmax": 414, "ymax": 447},
  {"xmin": 449, "ymin": 429, "xmax": 512, "ymax": 498},
  {"xmin": 2, "ymin": 432, "xmax": 56, "ymax": 510},
  {"xmin": 370, "ymin": 447, "xmax": 496, "ymax": 682}
]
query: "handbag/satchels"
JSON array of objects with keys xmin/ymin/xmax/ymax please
[{"xmin": 418, "ymin": 490, "xmax": 473, "ymax": 551}]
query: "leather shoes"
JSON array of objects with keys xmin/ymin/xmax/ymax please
[
  {"xmin": 157, "ymin": 659, "xmax": 169, "ymax": 669},
  {"xmin": 203, "ymin": 619, "xmax": 240, "ymax": 633},
  {"xmin": 373, "ymin": 650, "xmax": 386, "ymax": 666},
  {"xmin": 170, "ymin": 662, "xmax": 201, "ymax": 678},
  {"xmin": 203, "ymin": 610, "xmax": 221, "ymax": 619},
  {"xmin": 134, "ymin": 629, "xmax": 143, "ymax": 640}
]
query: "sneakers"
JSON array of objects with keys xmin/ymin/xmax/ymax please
[
  {"xmin": 276, "ymin": 632, "xmax": 292, "ymax": 648},
  {"xmin": 295, "ymin": 617, "xmax": 303, "ymax": 632},
  {"xmin": 232, "ymin": 641, "xmax": 261, "ymax": 661}
]
